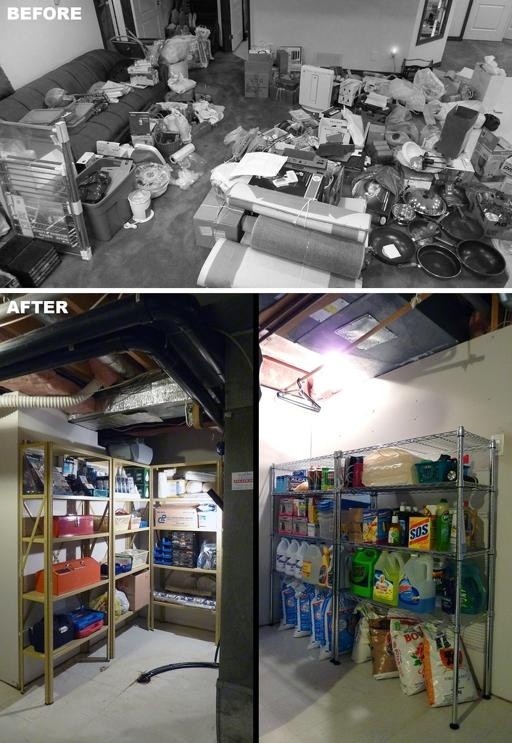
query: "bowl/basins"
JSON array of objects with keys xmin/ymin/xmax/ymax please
[{"xmin": 403, "ymin": 141, "xmax": 424, "ymax": 164}]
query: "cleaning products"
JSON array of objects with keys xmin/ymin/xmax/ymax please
[{"xmin": 319, "ymin": 544, "xmax": 334, "ymax": 586}]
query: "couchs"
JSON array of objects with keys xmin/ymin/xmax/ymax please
[{"xmin": 0, "ymin": 50, "xmax": 170, "ymax": 173}]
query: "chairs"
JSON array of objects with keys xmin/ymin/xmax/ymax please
[{"xmin": 111, "ymin": 36, "xmax": 147, "ymax": 59}]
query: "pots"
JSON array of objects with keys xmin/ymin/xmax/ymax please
[{"xmin": 366, "ymin": 187, "xmax": 507, "ymax": 280}]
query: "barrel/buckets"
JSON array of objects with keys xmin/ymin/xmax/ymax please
[
  {"xmin": 371, "ymin": 548, "xmax": 404, "ymax": 607},
  {"xmin": 295, "ymin": 540, "xmax": 309, "ymax": 579},
  {"xmin": 284, "ymin": 538, "xmax": 299, "ymax": 577},
  {"xmin": 449, "ymin": 557, "xmax": 487, "ymax": 615},
  {"xmin": 275, "ymin": 537, "xmax": 291, "ymax": 572},
  {"xmin": 350, "ymin": 546, "xmax": 378, "ymax": 599},
  {"xmin": 397, "ymin": 553, "xmax": 437, "ymax": 613},
  {"xmin": 301, "ymin": 544, "xmax": 322, "ymax": 584}
]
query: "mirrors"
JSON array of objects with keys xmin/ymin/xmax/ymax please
[{"xmin": 416, "ymin": 1, "xmax": 454, "ymax": 46}]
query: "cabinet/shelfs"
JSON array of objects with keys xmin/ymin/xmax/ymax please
[
  {"xmin": 146, "ymin": 459, "xmax": 221, "ymax": 644},
  {"xmin": 18, "ymin": 439, "xmax": 152, "ymax": 706},
  {"xmin": 271, "ymin": 426, "xmax": 497, "ymax": 730}
]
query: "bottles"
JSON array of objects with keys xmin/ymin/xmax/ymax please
[
  {"xmin": 308, "ymin": 464, "xmax": 334, "ymax": 491},
  {"xmin": 434, "ymin": 496, "xmax": 451, "ymax": 550},
  {"xmin": 317, "ymin": 502, "xmax": 332, "ymax": 536},
  {"xmin": 386, "ymin": 500, "xmax": 419, "ymax": 545},
  {"xmin": 353, "ymin": 455, "xmax": 363, "ymax": 487},
  {"xmin": 96, "ymin": 466, "xmax": 130, "ymax": 494}
]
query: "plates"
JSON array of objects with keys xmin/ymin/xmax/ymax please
[{"xmin": 396, "ymin": 150, "xmax": 446, "ymax": 172}]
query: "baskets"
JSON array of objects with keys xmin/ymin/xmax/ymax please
[{"xmin": 413, "ymin": 460, "xmax": 472, "ymax": 484}]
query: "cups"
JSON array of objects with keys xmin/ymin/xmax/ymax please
[{"xmin": 275, "ymin": 475, "xmax": 290, "ymax": 493}]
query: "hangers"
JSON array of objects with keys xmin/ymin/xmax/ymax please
[{"xmin": 277, "ymin": 376, "xmax": 322, "ymax": 414}]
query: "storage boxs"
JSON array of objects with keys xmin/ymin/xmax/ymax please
[
  {"xmin": 53, "ymin": 515, "xmax": 93, "ymax": 537},
  {"xmin": 74, "ymin": 54, "xmax": 512, "ymax": 251},
  {"xmin": 115, "ymin": 548, "xmax": 149, "ymax": 573},
  {"xmin": 155, "ymin": 503, "xmax": 218, "ymax": 526},
  {"xmin": 90, "ymin": 514, "xmax": 142, "ymax": 535},
  {"xmin": 118, "ymin": 570, "xmax": 151, "ymax": 613}
]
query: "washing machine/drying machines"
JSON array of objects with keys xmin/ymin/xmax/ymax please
[{"xmin": 157, "ymin": 472, "xmax": 168, "ymax": 498}]
